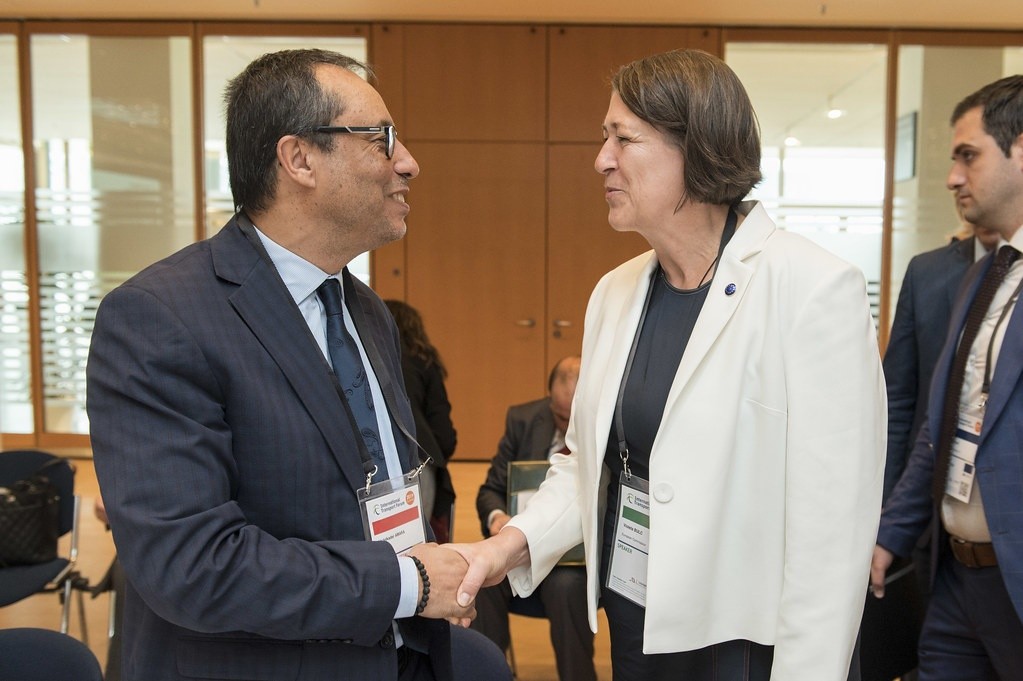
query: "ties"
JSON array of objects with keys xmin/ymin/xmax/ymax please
[
  {"xmin": 933, "ymin": 246, "xmax": 1021, "ymax": 496},
  {"xmin": 317, "ymin": 277, "xmax": 392, "ymax": 494}
]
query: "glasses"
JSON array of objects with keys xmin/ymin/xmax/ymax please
[{"xmin": 279, "ymin": 125, "xmax": 397, "ymax": 167}]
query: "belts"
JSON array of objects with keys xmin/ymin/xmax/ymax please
[{"xmin": 944, "ymin": 532, "xmax": 998, "ymax": 568}]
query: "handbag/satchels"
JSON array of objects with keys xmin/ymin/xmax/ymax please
[{"xmin": 0, "ymin": 475, "xmax": 60, "ymax": 567}]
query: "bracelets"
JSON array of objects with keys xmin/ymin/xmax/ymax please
[{"xmin": 406, "ymin": 555, "xmax": 431, "ymax": 618}]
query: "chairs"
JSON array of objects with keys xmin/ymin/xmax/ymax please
[
  {"xmin": 448, "ymin": 624, "xmax": 514, "ymax": 681},
  {"xmin": 0, "ymin": 627, "xmax": 103, "ymax": 681},
  {"xmin": 0, "ymin": 448, "xmax": 90, "ymax": 645}
]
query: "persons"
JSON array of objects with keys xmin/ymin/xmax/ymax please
[
  {"xmin": 439, "ymin": 51, "xmax": 889, "ymax": 680},
  {"xmin": 470, "ymin": 356, "xmax": 599, "ymax": 681},
  {"xmin": 861, "ymin": 76, "xmax": 1022, "ymax": 681},
  {"xmin": 385, "ymin": 295, "xmax": 512, "ymax": 681},
  {"xmin": 85, "ymin": 48, "xmax": 478, "ymax": 680}
]
